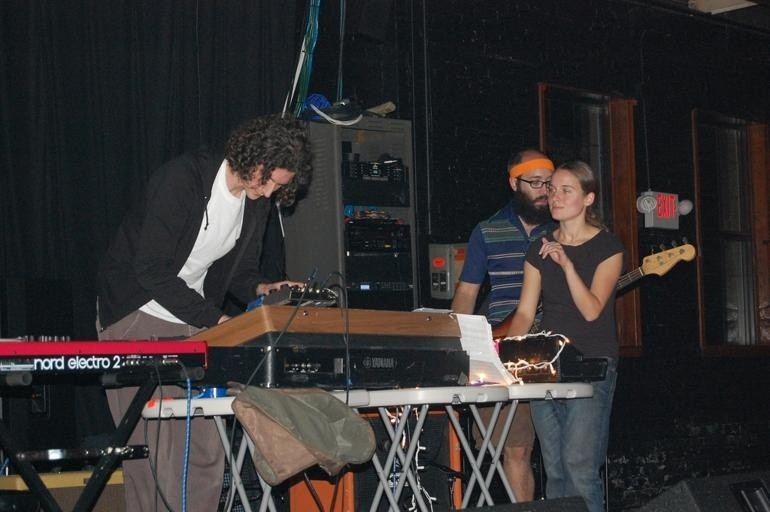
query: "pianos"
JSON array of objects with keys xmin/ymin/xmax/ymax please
[{"xmin": 0, "ymin": 335, "xmax": 210, "ymax": 389}]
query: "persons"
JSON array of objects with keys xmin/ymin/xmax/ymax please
[
  {"xmin": 450, "ymin": 148, "xmax": 560, "ymax": 504},
  {"xmin": 94, "ymin": 109, "xmax": 315, "ymax": 512},
  {"xmin": 504, "ymin": 160, "xmax": 627, "ymax": 511}
]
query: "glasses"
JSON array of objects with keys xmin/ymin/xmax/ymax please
[{"xmin": 516, "ymin": 176, "xmax": 552, "ymax": 189}]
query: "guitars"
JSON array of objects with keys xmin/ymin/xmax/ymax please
[{"xmin": 494, "ymin": 243, "xmax": 696, "ymax": 339}]
